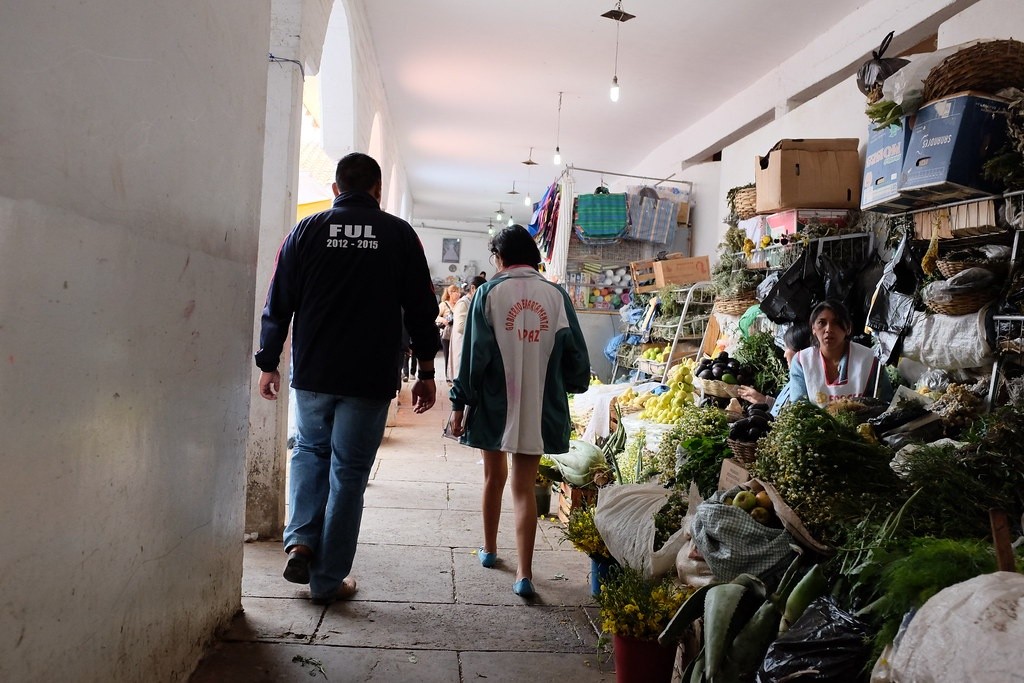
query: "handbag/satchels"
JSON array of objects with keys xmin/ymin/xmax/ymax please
[
  {"xmin": 435, "ymin": 302, "xmax": 453, "ymax": 329},
  {"xmin": 527, "ymin": 178, "xmax": 679, "ymax": 265}
]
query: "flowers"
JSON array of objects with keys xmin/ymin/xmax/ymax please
[
  {"xmin": 540, "ymin": 496, "xmax": 614, "ymax": 561},
  {"xmin": 587, "ymin": 557, "xmax": 698, "ymax": 662}
]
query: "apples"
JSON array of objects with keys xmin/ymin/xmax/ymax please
[
  {"xmin": 643, "ymin": 347, "xmax": 663, "ymax": 362},
  {"xmin": 645, "ymin": 367, "xmax": 694, "ymax": 425},
  {"xmin": 725, "ymin": 481, "xmax": 773, "ymax": 524}
]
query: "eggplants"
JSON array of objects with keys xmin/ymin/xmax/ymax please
[
  {"xmin": 695, "ymin": 351, "xmax": 755, "ymax": 407},
  {"xmin": 730, "ymin": 403, "xmax": 775, "ymax": 442}
]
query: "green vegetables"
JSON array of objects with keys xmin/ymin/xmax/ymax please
[
  {"xmin": 733, "ymin": 331, "xmax": 790, "ymax": 396},
  {"xmin": 862, "ymin": 530, "xmax": 1024, "ymax": 671}
]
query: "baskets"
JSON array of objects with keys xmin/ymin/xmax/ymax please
[
  {"xmin": 726, "ymin": 419, "xmax": 759, "ymax": 463},
  {"xmin": 734, "ymin": 189, "xmax": 758, "ymax": 220},
  {"xmin": 715, "ymin": 290, "xmax": 762, "ymax": 318},
  {"xmin": 922, "ymin": 38, "xmax": 1024, "ymax": 102},
  {"xmin": 936, "ymin": 261, "xmax": 971, "ymax": 279},
  {"xmin": 692, "ymin": 367, "xmax": 742, "ymax": 398},
  {"xmin": 921, "ymin": 267, "xmax": 993, "ymax": 316}
]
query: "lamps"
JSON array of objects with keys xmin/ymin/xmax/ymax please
[
  {"xmin": 508, "ymin": 181, "xmax": 519, "ymax": 227},
  {"xmin": 600, "ymin": 0, "xmax": 636, "ymax": 102},
  {"xmin": 488, "ymin": 201, "xmax": 505, "ymax": 234},
  {"xmin": 554, "ymin": 91, "xmax": 562, "ymax": 163},
  {"xmin": 521, "ymin": 148, "xmax": 539, "ymax": 206}
]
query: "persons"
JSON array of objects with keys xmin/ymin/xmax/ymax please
[
  {"xmin": 736, "ymin": 324, "xmax": 813, "ymax": 419},
  {"xmin": 442, "ymin": 224, "xmax": 590, "ymax": 598},
  {"xmin": 401, "ymin": 346, "xmax": 416, "ymax": 382},
  {"xmin": 444, "ymin": 276, "xmax": 488, "ymax": 387},
  {"xmin": 252, "ymin": 153, "xmax": 444, "ymax": 602},
  {"xmin": 787, "ymin": 298, "xmax": 893, "ymax": 407},
  {"xmin": 434, "ymin": 284, "xmax": 462, "ymax": 387},
  {"xmin": 480, "ymin": 271, "xmax": 486, "ymax": 279}
]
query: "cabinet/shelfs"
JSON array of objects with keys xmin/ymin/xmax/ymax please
[{"xmin": 610, "ymin": 189, "xmax": 1024, "ymax": 442}]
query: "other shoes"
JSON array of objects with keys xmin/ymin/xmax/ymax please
[
  {"xmin": 403, "ymin": 376, "xmax": 409, "ymax": 382},
  {"xmin": 410, "ymin": 374, "xmax": 415, "ymax": 380},
  {"xmin": 478, "ymin": 546, "xmax": 498, "ymax": 567},
  {"xmin": 512, "ymin": 578, "xmax": 535, "ymax": 596},
  {"xmin": 312, "ymin": 577, "xmax": 357, "ymax": 606},
  {"xmin": 283, "ymin": 546, "xmax": 314, "ymax": 584}
]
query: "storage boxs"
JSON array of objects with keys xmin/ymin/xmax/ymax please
[
  {"xmin": 896, "ymin": 90, "xmax": 1024, "ymax": 205},
  {"xmin": 718, "ymin": 457, "xmax": 751, "ymax": 493},
  {"xmin": 767, "ymin": 207, "xmax": 851, "ymax": 243},
  {"xmin": 860, "ymin": 108, "xmax": 924, "ymax": 211},
  {"xmin": 653, "ymin": 255, "xmax": 711, "ymax": 288},
  {"xmin": 754, "ymin": 139, "xmax": 863, "ymax": 213},
  {"xmin": 549, "ymin": 482, "xmax": 598, "ymax": 528}
]
